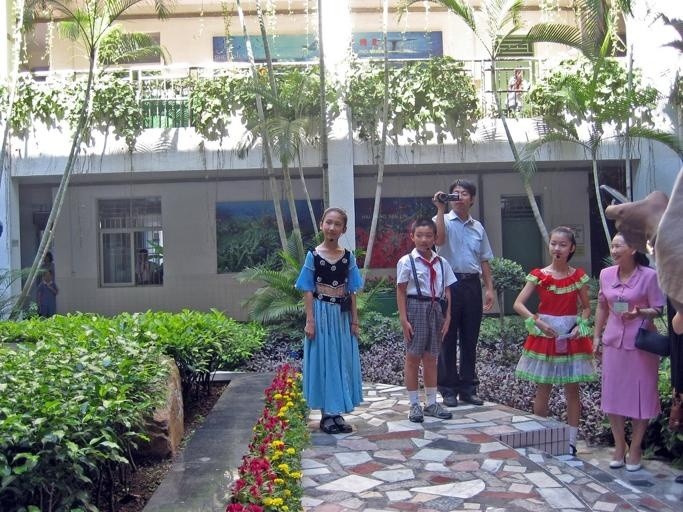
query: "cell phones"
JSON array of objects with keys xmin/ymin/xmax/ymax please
[{"xmin": 599, "ymin": 184, "xmax": 629, "ymax": 203}]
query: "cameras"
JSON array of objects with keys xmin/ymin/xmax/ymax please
[{"xmin": 437, "ymin": 193, "xmax": 459, "ymax": 202}]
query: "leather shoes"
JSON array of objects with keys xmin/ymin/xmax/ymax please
[
  {"xmin": 459, "ymin": 394, "xmax": 485, "ymax": 405},
  {"xmin": 443, "ymin": 396, "xmax": 457, "ymax": 406}
]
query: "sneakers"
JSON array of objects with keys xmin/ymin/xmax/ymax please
[
  {"xmin": 423, "ymin": 402, "xmax": 452, "ymax": 419},
  {"xmin": 409, "ymin": 405, "xmax": 425, "ymax": 422}
]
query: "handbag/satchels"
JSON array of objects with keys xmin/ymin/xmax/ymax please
[
  {"xmin": 669, "ymin": 398, "xmax": 683, "ymax": 433},
  {"xmin": 634, "ymin": 328, "xmax": 672, "ymax": 357}
]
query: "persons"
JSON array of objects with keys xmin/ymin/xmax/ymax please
[
  {"xmin": 507, "ymin": 68, "xmax": 528, "ymax": 109},
  {"xmin": 396, "ymin": 219, "xmax": 452, "ymax": 422},
  {"xmin": 294, "ymin": 207, "xmax": 364, "ymax": 433},
  {"xmin": 114, "ymin": 249, "xmax": 163, "ymax": 284},
  {"xmin": 607, "ymin": 163, "xmax": 683, "ymax": 483},
  {"xmin": 37, "ymin": 252, "xmax": 56, "ymax": 280},
  {"xmin": 593, "ymin": 231, "xmax": 665, "ymax": 471},
  {"xmin": 429, "ymin": 180, "xmax": 494, "ymax": 407},
  {"xmin": 512, "ymin": 226, "xmax": 597, "ymax": 458},
  {"xmin": 37, "ymin": 270, "xmax": 57, "ymax": 317}
]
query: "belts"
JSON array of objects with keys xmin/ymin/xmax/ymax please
[{"xmin": 456, "ymin": 273, "xmax": 479, "ymax": 280}]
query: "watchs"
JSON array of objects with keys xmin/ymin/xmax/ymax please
[
  {"xmin": 351, "ymin": 319, "xmax": 359, "ymax": 325},
  {"xmin": 645, "ymin": 232, "xmax": 657, "ymax": 256}
]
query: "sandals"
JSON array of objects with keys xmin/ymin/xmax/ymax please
[
  {"xmin": 334, "ymin": 415, "xmax": 352, "ymax": 433},
  {"xmin": 319, "ymin": 416, "xmax": 337, "ymax": 434}
]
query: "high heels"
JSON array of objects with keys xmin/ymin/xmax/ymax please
[
  {"xmin": 610, "ymin": 442, "xmax": 629, "ymax": 468},
  {"xmin": 625, "ymin": 449, "xmax": 642, "ymax": 471}
]
